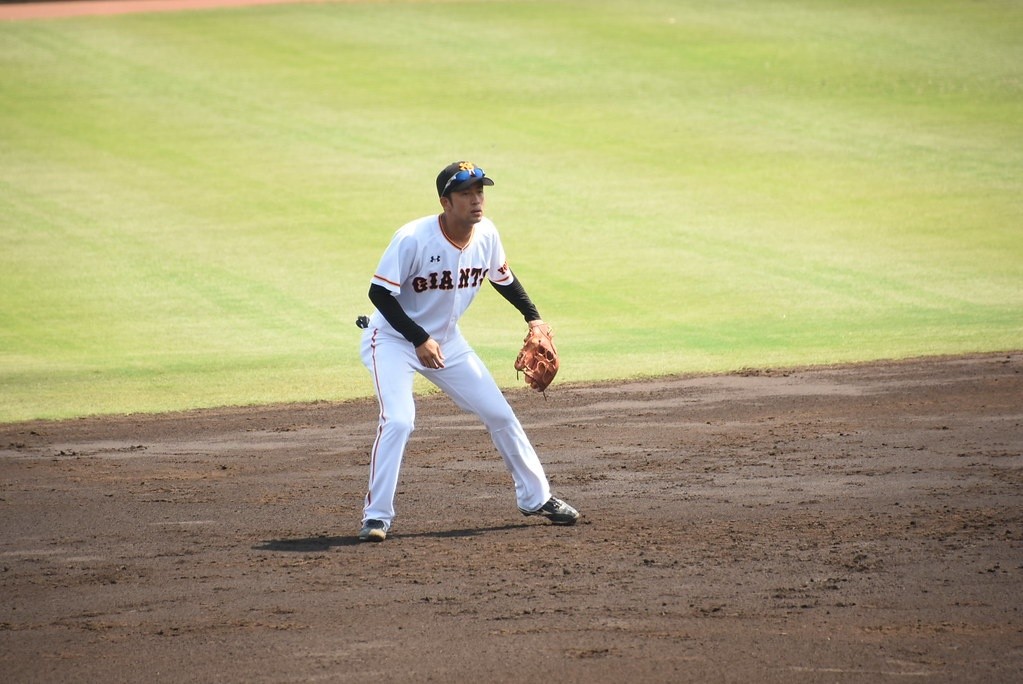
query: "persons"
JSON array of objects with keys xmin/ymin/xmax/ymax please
[{"xmin": 360, "ymin": 162, "xmax": 579, "ymax": 541}]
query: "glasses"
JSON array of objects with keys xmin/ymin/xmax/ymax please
[{"xmin": 441, "ymin": 168, "xmax": 485, "ymax": 197}]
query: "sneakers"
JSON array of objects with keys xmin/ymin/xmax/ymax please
[
  {"xmin": 517, "ymin": 497, "xmax": 579, "ymax": 524},
  {"xmin": 359, "ymin": 519, "xmax": 389, "ymax": 541}
]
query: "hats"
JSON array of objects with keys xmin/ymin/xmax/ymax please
[{"xmin": 436, "ymin": 161, "xmax": 495, "ymax": 198}]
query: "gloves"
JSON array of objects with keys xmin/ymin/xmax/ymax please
[{"xmin": 356, "ymin": 316, "xmax": 370, "ymax": 329}]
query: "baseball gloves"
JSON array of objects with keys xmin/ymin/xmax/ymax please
[{"xmin": 514, "ymin": 323, "xmax": 559, "ymax": 392}]
